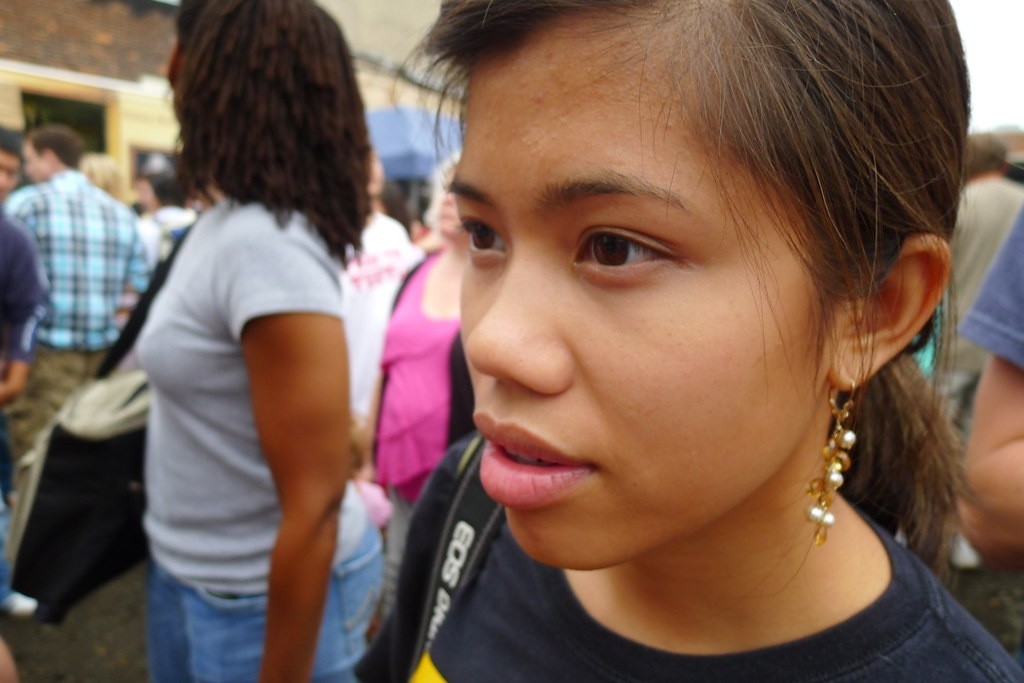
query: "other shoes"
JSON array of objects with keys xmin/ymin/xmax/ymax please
[{"xmin": 6, "ymin": 592, "xmax": 41, "ymax": 617}]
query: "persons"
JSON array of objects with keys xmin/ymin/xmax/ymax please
[
  {"xmin": 0, "ymin": 120, "xmax": 54, "ymax": 617},
  {"xmin": 956, "ymin": 194, "xmax": 1024, "ymax": 572},
  {"xmin": 81, "ymin": 150, "xmax": 199, "ymax": 262},
  {"xmin": 353, "ymin": 1, "xmax": 1023, "ymax": 683},
  {"xmin": 356, "ymin": 153, "xmax": 466, "ymax": 614},
  {"xmin": 932, "ymin": 131, "xmax": 1023, "ymax": 573},
  {"xmin": 131, "ymin": 0, "xmax": 382, "ymax": 682},
  {"xmin": 342, "ymin": 145, "xmax": 424, "ymax": 640},
  {"xmin": 5, "ymin": 123, "xmax": 152, "ymax": 606}
]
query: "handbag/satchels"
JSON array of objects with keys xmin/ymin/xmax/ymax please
[{"xmin": 3, "ymin": 218, "xmax": 153, "ymax": 624}]
